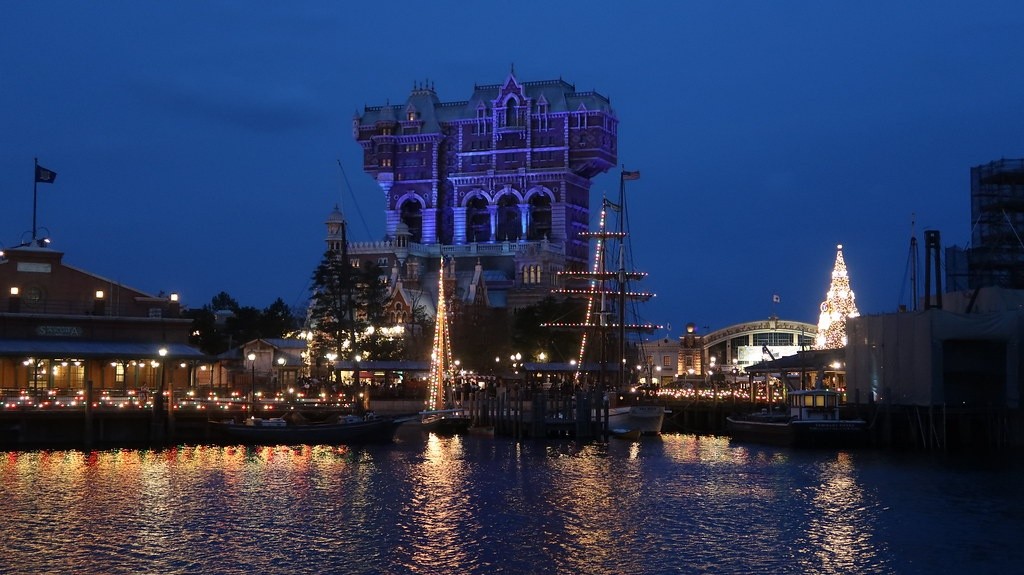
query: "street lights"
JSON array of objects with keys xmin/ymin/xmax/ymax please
[
  {"xmin": 158, "ymin": 347, "xmax": 168, "ymax": 411},
  {"xmin": 277, "ymin": 356, "xmax": 286, "ymax": 400},
  {"xmin": 247, "ymin": 353, "xmax": 256, "ymax": 413}
]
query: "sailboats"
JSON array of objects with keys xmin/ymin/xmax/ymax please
[{"xmin": 546, "ymin": 167, "xmax": 673, "ymax": 442}]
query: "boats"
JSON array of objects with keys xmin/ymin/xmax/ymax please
[
  {"xmin": 418, "ymin": 404, "xmax": 471, "ymax": 433},
  {"xmin": 724, "ymin": 355, "xmax": 868, "ymax": 448},
  {"xmin": 206, "ymin": 411, "xmax": 404, "ymax": 445}
]
